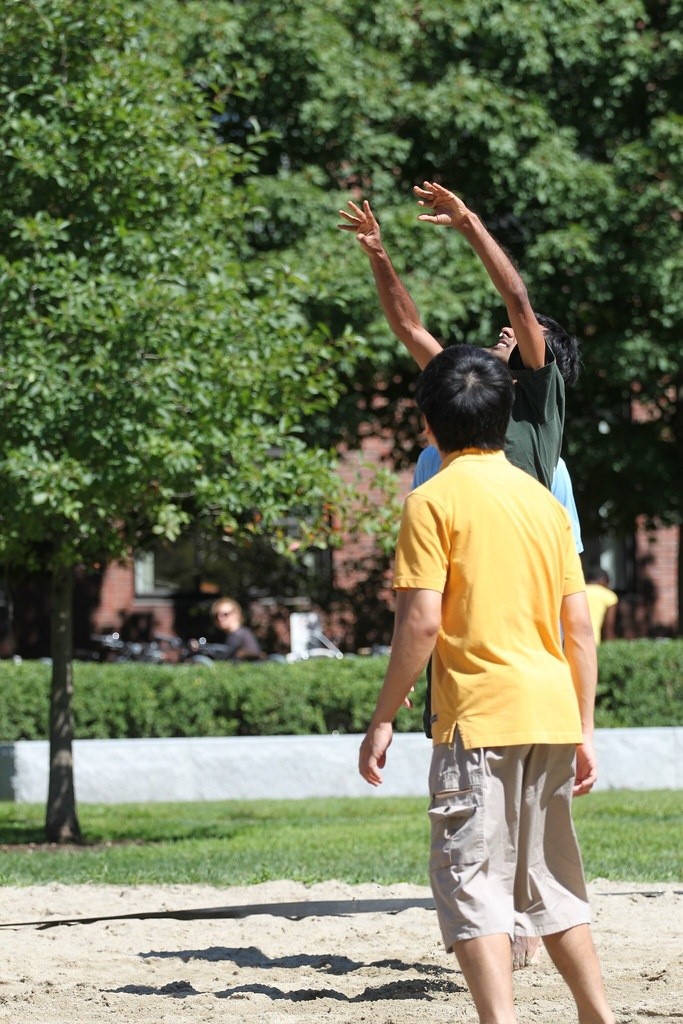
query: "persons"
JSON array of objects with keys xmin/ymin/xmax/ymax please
[
  {"xmin": 334, "ymin": 181, "xmax": 585, "ymax": 962},
  {"xmin": 177, "ymin": 595, "xmax": 266, "ymax": 664},
  {"xmin": 357, "ymin": 343, "xmax": 626, "ymax": 1024}
]
